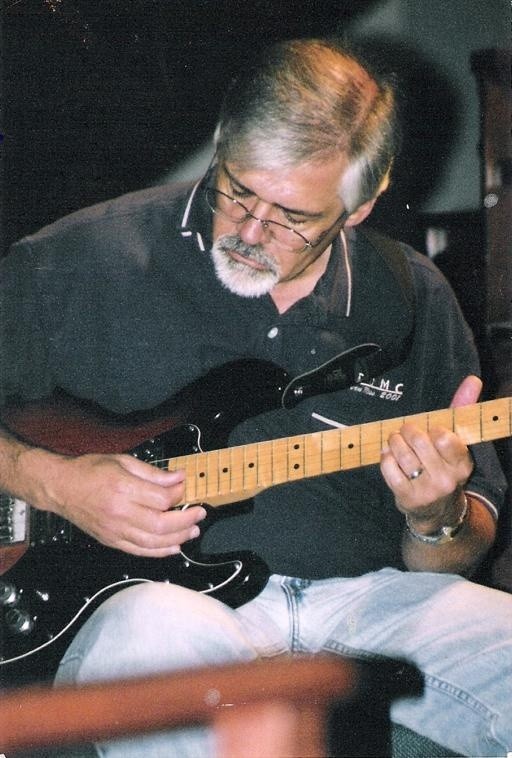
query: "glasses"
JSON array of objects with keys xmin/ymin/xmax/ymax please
[{"xmin": 200, "ymin": 150, "xmax": 347, "ymax": 254}]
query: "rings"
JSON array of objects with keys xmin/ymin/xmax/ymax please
[{"xmin": 410, "ymin": 468, "xmax": 426, "ymax": 485}]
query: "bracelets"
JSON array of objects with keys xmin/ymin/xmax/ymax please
[{"xmin": 404, "ymin": 491, "xmax": 470, "ymax": 547}]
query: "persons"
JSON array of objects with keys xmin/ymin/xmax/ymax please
[{"xmin": 1, "ymin": 37, "xmax": 510, "ymax": 756}]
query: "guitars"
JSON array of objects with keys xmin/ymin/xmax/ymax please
[{"xmin": 0, "ymin": 355, "xmax": 512, "ymax": 662}]
